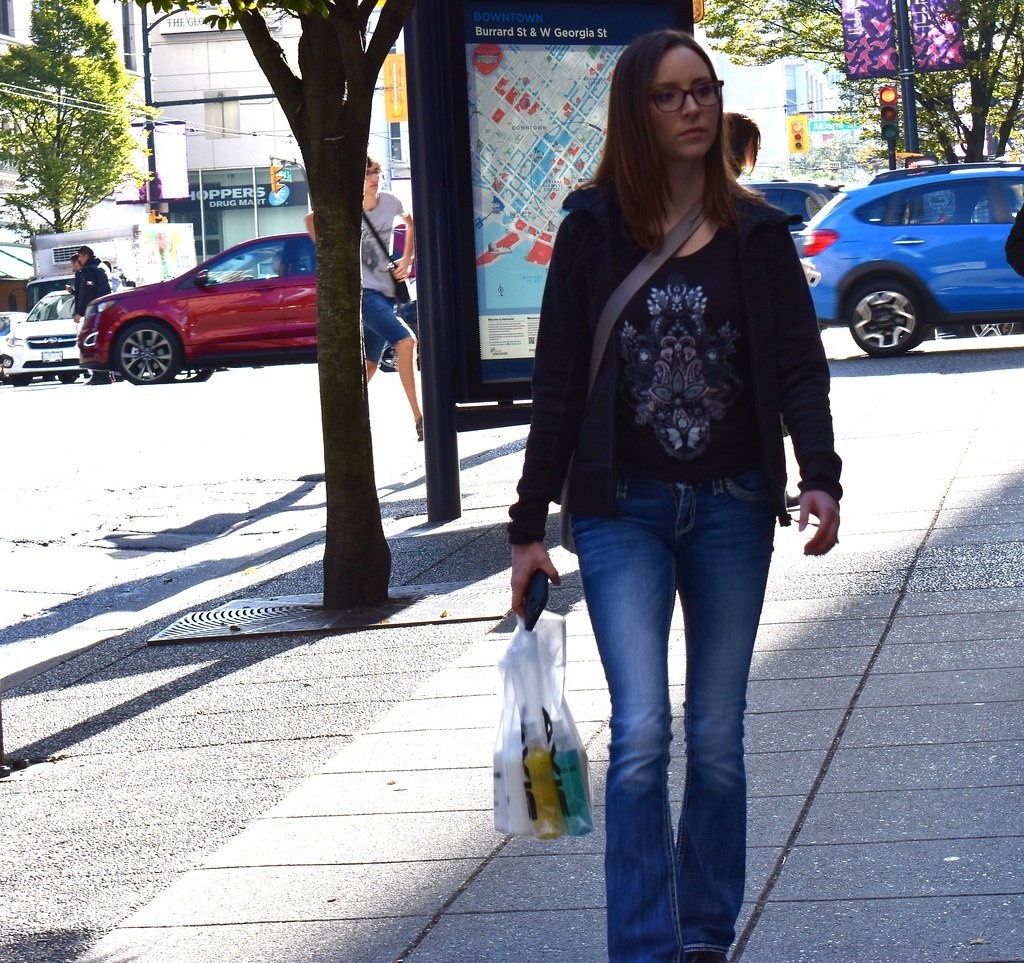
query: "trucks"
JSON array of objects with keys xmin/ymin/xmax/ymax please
[{"xmin": 26, "ymin": 222, "xmax": 197, "ymax": 315}]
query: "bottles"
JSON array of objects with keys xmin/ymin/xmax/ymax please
[{"xmin": 490, "ymin": 717, "xmax": 593, "ymax": 839}]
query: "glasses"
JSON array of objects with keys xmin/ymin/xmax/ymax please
[
  {"xmin": 648, "ymin": 80, "xmax": 724, "ymax": 113},
  {"xmin": 366, "ymin": 169, "xmax": 381, "ymax": 176}
]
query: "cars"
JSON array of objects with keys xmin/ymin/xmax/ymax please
[
  {"xmin": 0, "ymin": 285, "xmax": 133, "ymax": 387},
  {"xmin": 76, "ymin": 223, "xmax": 420, "ymax": 385}
]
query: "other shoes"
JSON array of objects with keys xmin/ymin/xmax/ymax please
[
  {"xmin": 786, "ymin": 494, "xmax": 801, "ymax": 508},
  {"xmin": 685, "ymin": 951, "xmax": 727, "ymax": 963},
  {"xmin": 84, "ymin": 377, "xmax": 112, "ymax": 385},
  {"xmin": 559, "ymin": 450, "xmax": 576, "ymax": 554},
  {"xmin": 417, "ymin": 419, "xmax": 423, "ymax": 441}
]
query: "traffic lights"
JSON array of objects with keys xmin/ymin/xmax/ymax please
[
  {"xmin": 787, "ymin": 114, "xmax": 810, "ymax": 155},
  {"xmin": 270, "ymin": 165, "xmax": 285, "ymax": 194},
  {"xmin": 880, "ymin": 87, "xmax": 901, "ymax": 141}
]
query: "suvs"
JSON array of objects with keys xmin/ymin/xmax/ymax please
[
  {"xmin": 795, "ymin": 162, "xmax": 1024, "ymax": 357},
  {"xmin": 742, "ymin": 178, "xmax": 837, "ymax": 235}
]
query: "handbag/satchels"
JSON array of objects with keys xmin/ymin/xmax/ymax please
[
  {"xmin": 493, "ymin": 608, "xmax": 592, "ymax": 839},
  {"xmin": 390, "ymin": 265, "xmax": 410, "ymax": 304}
]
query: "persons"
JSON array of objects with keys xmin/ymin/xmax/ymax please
[
  {"xmin": 505, "ymin": 27, "xmax": 843, "ymax": 963},
  {"xmin": 65, "ymin": 245, "xmax": 136, "ymax": 386},
  {"xmin": 303, "ymin": 156, "xmax": 424, "ymax": 443}
]
